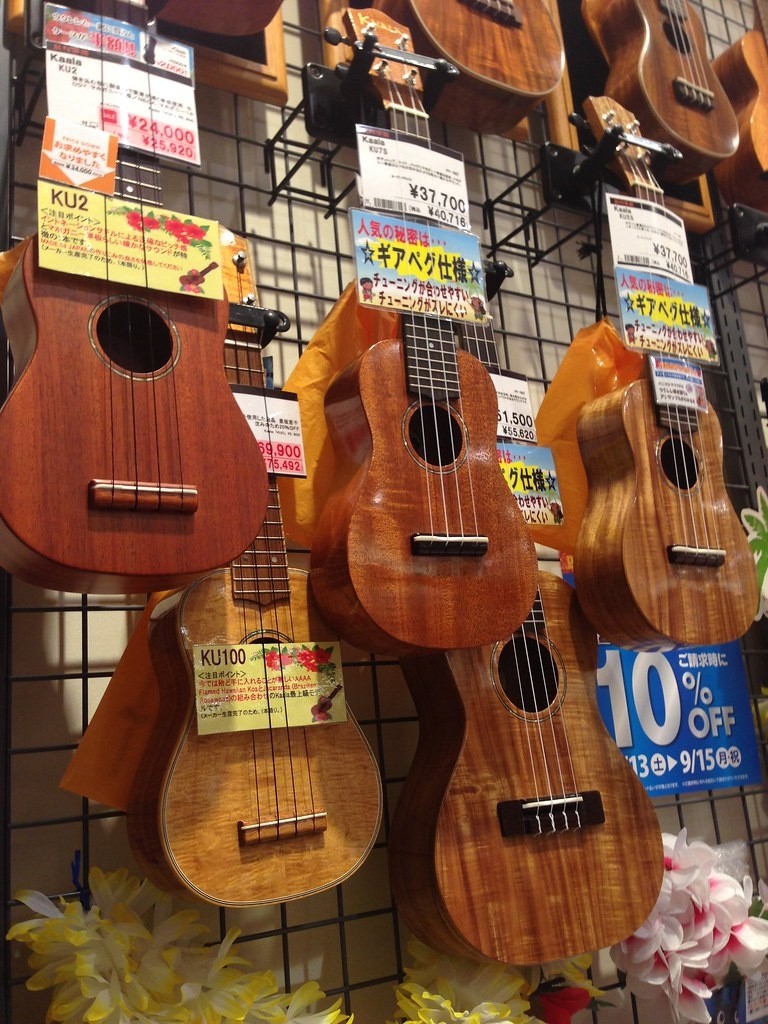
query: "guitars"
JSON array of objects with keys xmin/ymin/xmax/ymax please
[
  {"xmin": 0, "ymin": 0, "xmax": 270, "ymax": 588},
  {"xmin": 387, "ymin": 255, "xmax": 665, "ymax": 968},
  {"xmin": 128, "ymin": 225, "xmax": 383, "ymax": 905},
  {"xmin": 367, "ymin": 0, "xmax": 768, "ymax": 212},
  {"xmin": 542, "ymin": 93, "xmax": 759, "ymax": 652},
  {"xmin": 309, "ymin": 8, "xmax": 538, "ymax": 658}
]
